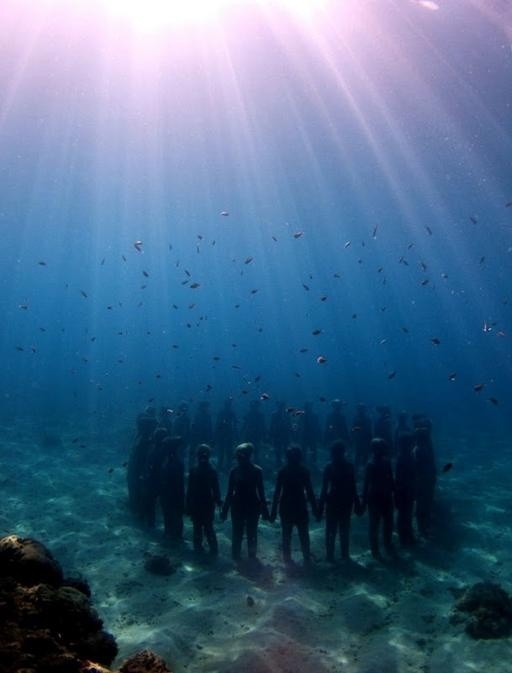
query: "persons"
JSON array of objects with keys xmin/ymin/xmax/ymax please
[{"xmin": 127, "ymin": 397, "xmax": 437, "ymax": 565}]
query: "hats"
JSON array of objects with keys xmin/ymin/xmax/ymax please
[
  {"xmin": 195, "ymin": 443, "xmax": 211, "ymax": 458},
  {"xmin": 234, "ymin": 442, "xmax": 255, "ymax": 461}
]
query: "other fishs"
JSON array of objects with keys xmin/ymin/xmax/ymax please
[{"xmin": 0, "ymin": 211, "xmax": 511, "ymax": 506}]
